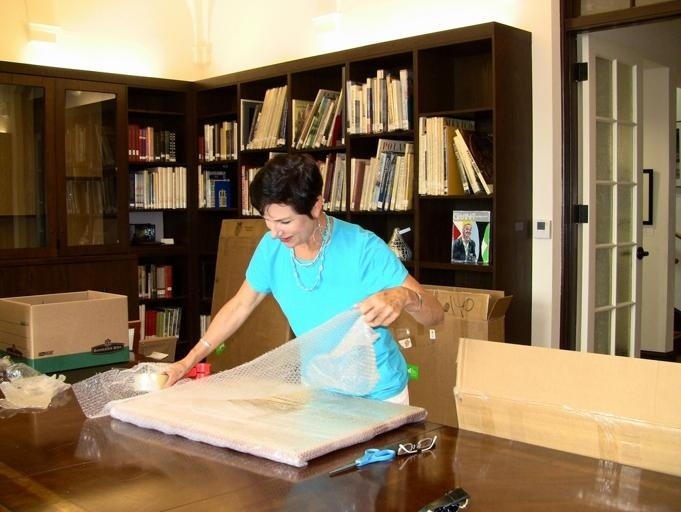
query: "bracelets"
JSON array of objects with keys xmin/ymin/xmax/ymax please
[
  {"xmin": 199, "ymin": 336, "xmax": 211, "ymax": 351},
  {"xmin": 416, "ymin": 292, "xmax": 429, "ymax": 311}
]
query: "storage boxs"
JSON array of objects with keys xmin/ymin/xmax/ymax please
[
  {"xmin": 388, "ymin": 284, "xmax": 513, "ymax": 429},
  {"xmin": 453, "ymin": 338, "xmax": 681, "ymax": 477},
  {"xmin": 0, "ymin": 290, "xmax": 129, "ymax": 374},
  {"xmin": 206, "ymin": 219, "xmax": 296, "ymax": 375}
]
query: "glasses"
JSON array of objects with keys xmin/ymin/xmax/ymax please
[{"xmin": 396, "ymin": 435, "xmax": 438, "ymax": 457}]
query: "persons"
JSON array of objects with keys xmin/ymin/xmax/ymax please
[
  {"xmin": 454, "ymin": 224, "xmax": 478, "ymax": 260},
  {"xmin": 159, "ymin": 151, "xmax": 446, "ymax": 403}
]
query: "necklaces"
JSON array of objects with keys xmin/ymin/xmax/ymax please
[{"xmin": 291, "ymin": 218, "xmax": 333, "ymax": 292}]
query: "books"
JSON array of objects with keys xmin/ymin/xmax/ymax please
[
  {"xmin": 451, "ymin": 211, "xmax": 491, "ymax": 264},
  {"xmin": 130, "ymin": 124, "xmax": 186, "ymax": 209},
  {"xmin": 199, "ymin": 68, "xmax": 413, "ymax": 216},
  {"xmin": 419, "ymin": 116, "xmax": 495, "ymax": 195},
  {"xmin": 132, "ymin": 218, "xmax": 215, "ymax": 363}
]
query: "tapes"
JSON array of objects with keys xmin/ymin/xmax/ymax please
[{"xmin": 133, "ymin": 373, "xmax": 168, "ymax": 392}]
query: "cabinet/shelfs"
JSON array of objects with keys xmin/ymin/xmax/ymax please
[
  {"xmin": 196, "ymin": 21, "xmax": 533, "ymax": 363},
  {"xmin": 2, "ymin": 60, "xmax": 196, "ymax": 363}
]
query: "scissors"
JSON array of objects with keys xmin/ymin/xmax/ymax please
[{"xmin": 329, "ymin": 448, "xmax": 396, "ymax": 477}]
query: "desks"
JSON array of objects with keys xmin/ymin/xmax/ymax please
[{"xmin": 0, "ymin": 350, "xmax": 681, "ymax": 512}]
